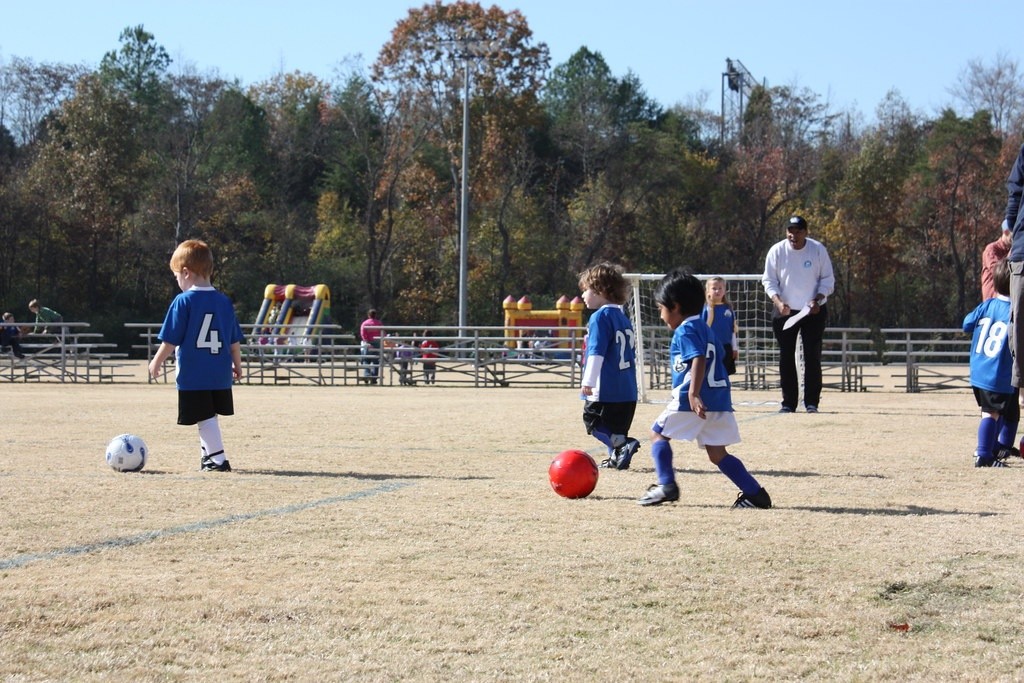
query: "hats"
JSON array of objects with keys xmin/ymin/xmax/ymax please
[
  {"xmin": 1002, "ymin": 220, "xmax": 1009, "ymax": 231},
  {"xmin": 787, "ymin": 216, "xmax": 808, "ymax": 229}
]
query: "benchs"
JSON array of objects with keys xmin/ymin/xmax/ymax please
[{"xmin": 1, "ymin": 321, "xmax": 973, "ymax": 392}]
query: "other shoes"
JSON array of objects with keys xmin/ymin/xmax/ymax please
[
  {"xmin": 779, "ymin": 406, "xmax": 793, "ymax": 413},
  {"xmin": 807, "ymin": 406, "xmax": 818, "ymax": 413},
  {"xmin": 202, "ymin": 457, "xmax": 230, "ymax": 471},
  {"xmin": 998, "ymin": 441, "xmax": 1020, "ymax": 456}
]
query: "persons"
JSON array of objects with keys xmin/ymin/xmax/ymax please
[
  {"xmin": 516, "ymin": 331, "xmax": 557, "ymax": 364},
  {"xmin": 761, "ymin": 216, "xmax": 835, "ymax": 414},
  {"xmin": 699, "ymin": 276, "xmax": 739, "ymax": 410},
  {"xmin": 250, "ymin": 301, "xmax": 313, "ymax": 362},
  {"xmin": 0, "ymin": 312, "xmax": 28, "ymax": 360},
  {"xmin": 576, "ymin": 262, "xmax": 642, "ymax": 470},
  {"xmin": 360, "ymin": 309, "xmax": 439, "ymax": 386},
  {"xmin": 963, "ymin": 144, "xmax": 1024, "ymax": 468},
  {"xmin": 149, "ymin": 240, "xmax": 244, "ymax": 474},
  {"xmin": 637, "ymin": 270, "xmax": 772, "ymax": 510},
  {"xmin": 28, "ymin": 299, "xmax": 76, "ymax": 354}
]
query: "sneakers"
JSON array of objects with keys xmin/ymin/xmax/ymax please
[
  {"xmin": 637, "ymin": 482, "xmax": 679, "ymax": 505},
  {"xmin": 730, "ymin": 487, "xmax": 771, "ymax": 509},
  {"xmin": 598, "ymin": 457, "xmax": 616, "ymax": 468},
  {"xmin": 975, "ymin": 455, "xmax": 1008, "ymax": 468},
  {"xmin": 974, "ymin": 444, "xmax": 1010, "ymax": 459},
  {"xmin": 615, "ymin": 436, "xmax": 641, "ymax": 470}
]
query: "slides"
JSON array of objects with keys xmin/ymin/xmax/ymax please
[{"xmin": 250, "ymin": 300, "xmax": 324, "ymax": 355}]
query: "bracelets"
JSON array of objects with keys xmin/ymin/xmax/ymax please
[{"xmin": 815, "ymin": 299, "xmax": 822, "ymax": 304}]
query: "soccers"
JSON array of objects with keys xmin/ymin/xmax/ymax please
[
  {"xmin": 106, "ymin": 434, "xmax": 147, "ymax": 472},
  {"xmin": 548, "ymin": 450, "xmax": 598, "ymax": 498}
]
